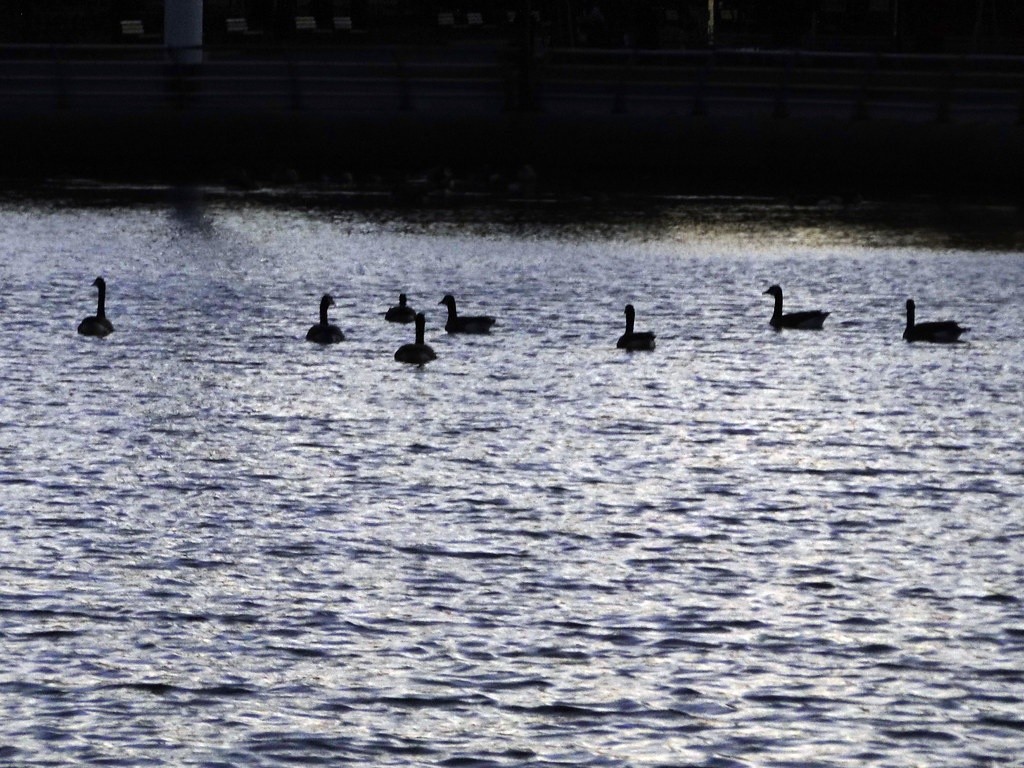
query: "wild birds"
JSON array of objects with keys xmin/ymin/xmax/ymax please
[
  {"xmin": 437, "ymin": 294, "xmax": 495, "ymax": 336},
  {"xmin": 616, "ymin": 304, "xmax": 657, "ymax": 354},
  {"xmin": 903, "ymin": 299, "xmax": 970, "ymax": 343},
  {"xmin": 306, "ymin": 293, "xmax": 345, "ymax": 346},
  {"xmin": 394, "ymin": 313, "xmax": 438, "ymax": 368},
  {"xmin": 762, "ymin": 284, "xmax": 832, "ymax": 334},
  {"xmin": 384, "ymin": 294, "xmax": 416, "ymax": 324},
  {"xmin": 78, "ymin": 276, "xmax": 114, "ymax": 338}
]
